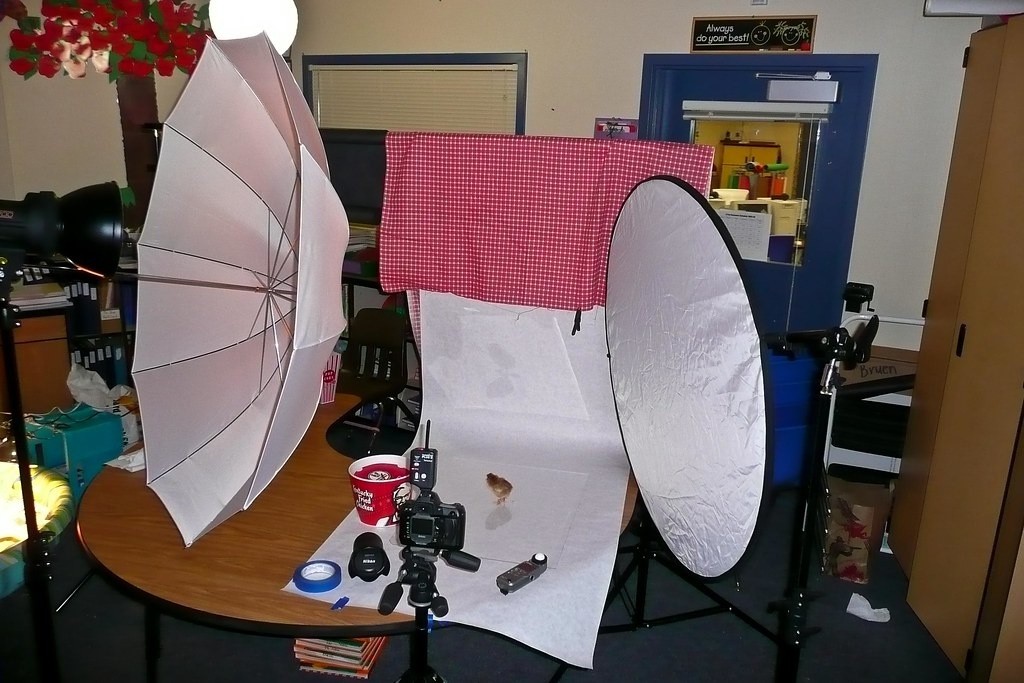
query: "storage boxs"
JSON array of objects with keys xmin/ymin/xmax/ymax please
[{"xmin": 25, "ymin": 407, "xmax": 123, "ymax": 519}]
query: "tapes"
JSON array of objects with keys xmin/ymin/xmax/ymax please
[{"xmin": 293, "ymin": 560, "xmax": 342, "ymax": 593}]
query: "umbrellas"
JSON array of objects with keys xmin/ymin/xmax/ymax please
[{"xmin": 88, "ymin": 30, "xmax": 350, "ymax": 547}]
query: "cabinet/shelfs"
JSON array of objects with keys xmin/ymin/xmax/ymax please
[
  {"xmin": 0, "ymin": 299, "xmax": 75, "ymax": 439},
  {"xmin": 886, "ymin": 14, "xmax": 1024, "ymax": 683}
]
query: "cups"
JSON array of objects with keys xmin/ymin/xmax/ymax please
[{"xmin": 348, "ymin": 454, "xmax": 412, "ymax": 527}]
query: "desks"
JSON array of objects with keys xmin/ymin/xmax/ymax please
[
  {"xmin": 77, "ymin": 393, "xmax": 640, "ymax": 683},
  {"xmin": 340, "ymin": 248, "xmax": 415, "ymax": 344}
]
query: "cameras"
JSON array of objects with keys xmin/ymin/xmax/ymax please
[{"xmin": 399, "ymin": 492, "xmax": 465, "ymax": 550}]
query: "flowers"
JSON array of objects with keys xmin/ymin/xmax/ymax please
[{"xmin": 8, "ymin": 0, "xmax": 217, "ymax": 84}]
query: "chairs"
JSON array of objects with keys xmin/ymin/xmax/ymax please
[{"xmin": 335, "ymin": 308, "xmax": 408, "ymax": 402}]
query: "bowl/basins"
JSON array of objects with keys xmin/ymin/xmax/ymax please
[{"xmin": 713, "ymin": 188, "xmax": 749, "ymax": 200}]
